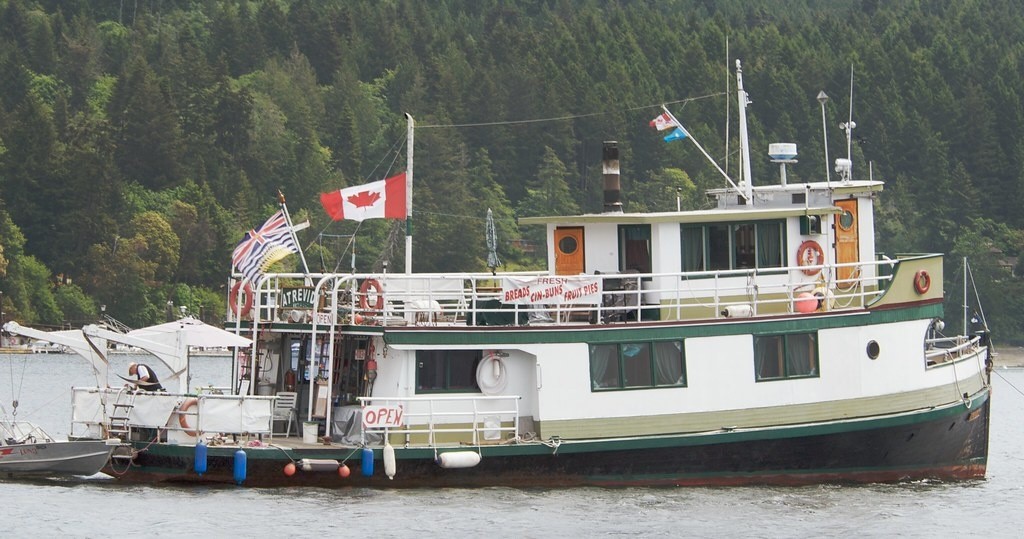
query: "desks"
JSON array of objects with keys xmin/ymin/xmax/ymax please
[{"xmin": 467, "ymin": 300, "xmax": 529, "ymax": 328}]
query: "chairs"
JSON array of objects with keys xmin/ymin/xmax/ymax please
[{"xmin": 267, "ymin": 392, "xmax": 299, "ymax": 438}]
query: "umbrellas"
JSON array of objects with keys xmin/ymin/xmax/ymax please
[{"xmin": 485, "ymin": 208, "xmax": 502, "ymax": 288}]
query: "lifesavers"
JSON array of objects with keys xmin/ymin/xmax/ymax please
[
  {"xmin": 179, "ymin": 397, "xmax": 206, "ymax": 438},
  {"xmin": 357, "ymin": 278, "xmax": 385, "ymax": 316},
  {"xmin": 229, "ymin": 282, "xmax": 255, "ymax": 316},
  {"xmin": 796, "ymin": 240, "xmax": 825, "ymax": 275},
  {"xmin": 914, "ymin": 269, "xmax": 931, "ymax": 294}
]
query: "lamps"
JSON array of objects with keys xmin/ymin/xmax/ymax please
[{"xmin": 290, "ymin": 310, "xmax": 306, "ymax": 322}]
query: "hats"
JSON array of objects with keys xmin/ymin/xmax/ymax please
[{"xmin": 127, "ymin": 362, "xmax": 137, "ymax": 376}]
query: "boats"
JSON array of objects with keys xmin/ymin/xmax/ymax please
[
  {"xmin": 0, "ymin": 421, "xmax": 121, "ymax": 476},
  {"xmin": 70, "ymin": 35, "xmax": 994, "ymax": 488}
]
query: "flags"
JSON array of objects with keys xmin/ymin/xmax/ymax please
[
  {"xmin": 320, "ymin": 172, "xmax": 407, "ymax": 221},
  {"xmin": 232, "ymin": 209, "xmax": 299, "ymax": 285},
  {"xmin": 649, "ymin": 113, "xmax": 687, "ymax": 143}
]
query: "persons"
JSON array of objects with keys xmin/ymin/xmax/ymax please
[{"xmin": 125, "ymin": 362, "xmax": 163, "ymax": 391}]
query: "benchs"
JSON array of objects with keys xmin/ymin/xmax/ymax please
[{"xmin": 548, "ymin": 272, "xmax": 629, "ymax": 323}]
